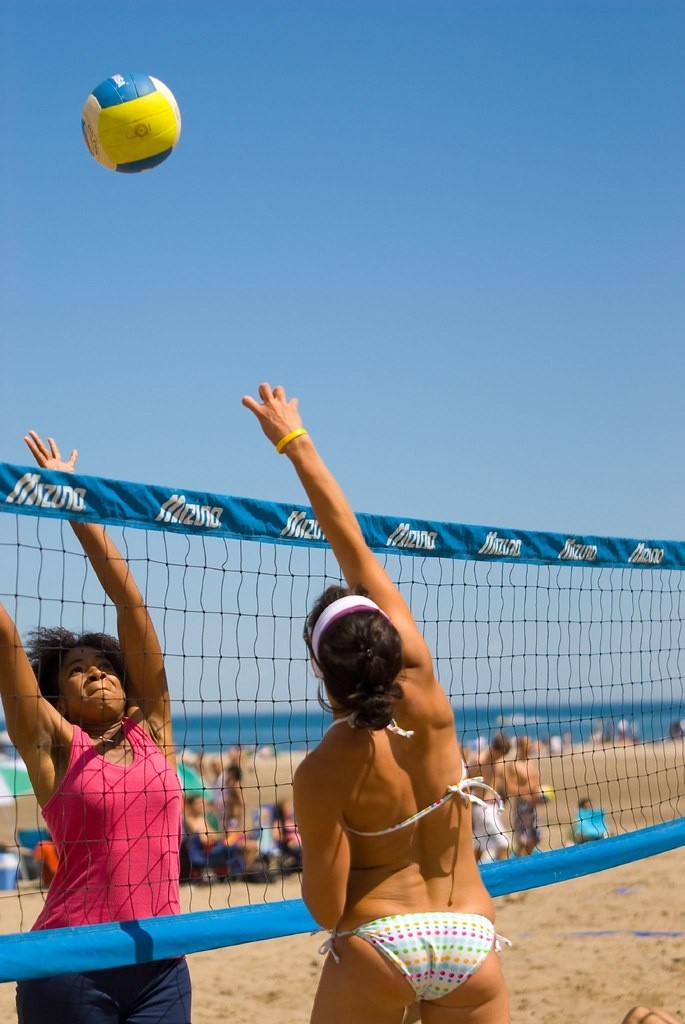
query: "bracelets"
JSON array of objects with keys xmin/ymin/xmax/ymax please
[{"xmin": 275, "ymin": 427, "xmax": 307, "ymax": 454}]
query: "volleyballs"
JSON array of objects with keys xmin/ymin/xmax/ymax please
[{"xmin": 81, "ymin": 71, "xmax": 184, "ymax": 176}]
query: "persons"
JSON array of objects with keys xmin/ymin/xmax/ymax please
[
  {"xmin": 0, "ymin": 427, "xmax": 193, "ymax": 1024},
  {"xmin": 243, "ymin": 381, "xmax": 514, "ymax": 1024},
  {"xmin": 184, "ymin": 713, "xmax": 685, "ymax": 882}
]
